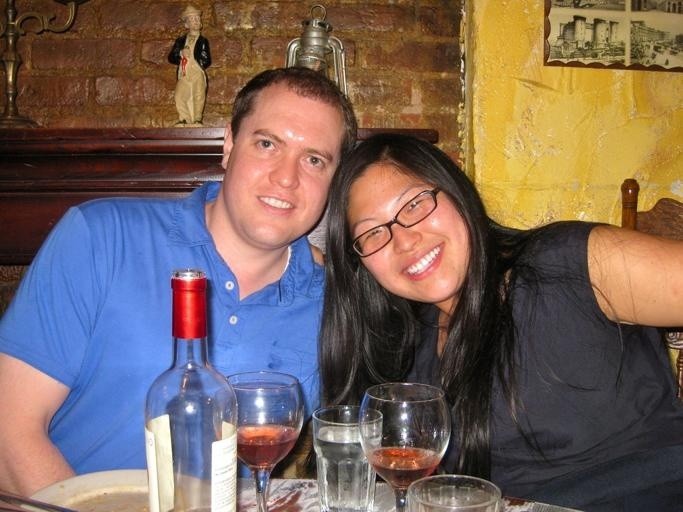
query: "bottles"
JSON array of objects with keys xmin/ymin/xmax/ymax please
[{"xmin": 142, "ymin": 268, "xmax": 240, "ymax": 512}]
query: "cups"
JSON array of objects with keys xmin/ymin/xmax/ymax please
[
  {"xmin": 311, "ymin": 404, "xmax": 385, "ymax": 512},
  {"xmin": 406, "ymin": 473, "xmax": 502, "ymax": 512}
]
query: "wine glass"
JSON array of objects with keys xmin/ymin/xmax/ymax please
[
  {"xmin": 211, "ymin": 371, "xmax": 305, "ymax": 512},
  {"xmin": 356, "ymin": 381, "xmax": 453, "ymax": 512}
]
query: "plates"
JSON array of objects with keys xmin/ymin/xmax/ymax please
[{"xmin": 19, "ymin": 467, "xmax": 210, "ymax": 512}]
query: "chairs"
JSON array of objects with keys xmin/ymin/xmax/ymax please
[{"xmin": 621, "ymin": 178, "xmax": 683, "ymax": 401}]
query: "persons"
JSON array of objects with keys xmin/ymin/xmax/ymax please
[
  {"xmin": 318, "ymin": 133, "xmax": 683, "ymax": 512},
  {"xmin": 0, "ymin": 65, "xmax": 374, "ymax": 511},
  {"xmin": 166, "ymin": 3, "xmax": 211, "ymax": 127}
]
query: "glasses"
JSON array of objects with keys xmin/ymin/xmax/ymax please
[{"xmin": 350, "ymin": 186, "xmax": 442, "ymax": 259}]
